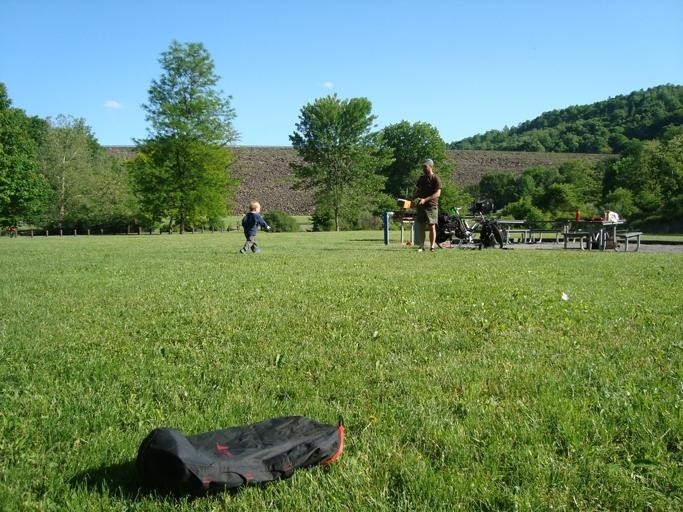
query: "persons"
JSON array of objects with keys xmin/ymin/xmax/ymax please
[
  {"xmin": 239, "ymin": 201, "xmax": 270, "ymax": 256},
  {"xmin": 414, "ymin": 156, "xmax": 442, "ymax": 253}
]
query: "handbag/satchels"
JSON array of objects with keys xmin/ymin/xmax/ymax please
[
  {"xmin": 587, "ymin": 231, "xmax": 618, "ymax": 249},
  {"xmin": 436, "ymin": 218, "xmax": 458, "ymax": 244}
]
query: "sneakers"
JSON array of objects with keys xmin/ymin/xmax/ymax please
[
  {"xmin": 415, "ymin": 247, "xmax": 425, "ymax": 252},
  {"xmin": 430, "ymin": 248, "xmax": 436, "ymax": 253},
  {"xmin": 253, "ymin": 248, "xmax": 263, "ymax": 254},
  {"xmin": 239, "ymin": 249, "xmax": 246, "ymax": 254}
]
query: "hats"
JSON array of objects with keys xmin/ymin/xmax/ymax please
[{"xmin": 421, "ymin": 159, "xmax": 433, "ymax": 167}]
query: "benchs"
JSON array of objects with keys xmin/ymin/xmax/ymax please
[{"xmin": 400, "ymin": 219, "xmax": 645, "ymax": 251}]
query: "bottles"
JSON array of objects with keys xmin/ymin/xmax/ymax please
[
  {"xmin": 604, "ymin": 209, "xmax": 610, "ymax": 223},
  {"xmin": 575, "ymin": 208, "xmax": 581, "ymax": 222}
]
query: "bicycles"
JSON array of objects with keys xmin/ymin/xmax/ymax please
[{"xmin": 435, "ymin": 197, "xmax": 507, "ymax": 250}]
